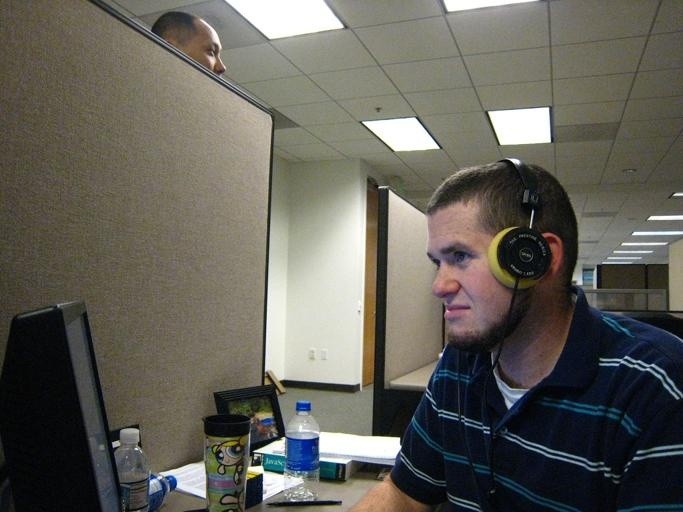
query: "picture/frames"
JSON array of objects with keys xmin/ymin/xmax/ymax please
[{"xmin": 214, "ymin": 384, "xmax": 285, "ymax": 456}]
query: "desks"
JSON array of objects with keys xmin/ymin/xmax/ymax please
[
  {"xmin": 389, "ymin": 355, "xmax": 439, "ymax": 391},
  {"xmin": 151, "ymin": 459, "xmax": 381, "ymax": 512}
]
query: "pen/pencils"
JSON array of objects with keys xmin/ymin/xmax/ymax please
[{"xmin": 265, "ymin": 501, "xmax": 342, "ymax": 506}]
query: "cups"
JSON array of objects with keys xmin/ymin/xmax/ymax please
[{"xmin": 201, "ymin": 412, "xmax": 252, "ymax": 512}]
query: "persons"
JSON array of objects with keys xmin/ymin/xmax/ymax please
[
  {"xmin": 333, "ymin": 155, "xmax": 683, "ymax": 512},
  {"xmin": 150, "ymin": 10, "xmax": 227, "ymax": 78}
]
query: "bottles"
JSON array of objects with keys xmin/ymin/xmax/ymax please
[
  {"xmin": 111, "ymin": 425, "xmax": 148, "ymax": 510},
  {"xmin": 147, "ymin": 469, "xmax": 179, "ymax": 511},
  {"xmin": 280, "ymin": 399, "xmax": 324, "ymax": 504}
]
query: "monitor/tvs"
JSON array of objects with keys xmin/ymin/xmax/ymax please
[{"xmin": 0, "ymin": 299, "xmax": 124, "ymax": 512}]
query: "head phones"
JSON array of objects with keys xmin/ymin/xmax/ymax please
[{"xmin": 487, "ymin": 157, "xmax": 554, "ymax": 292}]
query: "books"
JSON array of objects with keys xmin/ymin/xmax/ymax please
[{"xmin": 250, "ymin": 438, "xmax": 369, "ymax": 482}]
